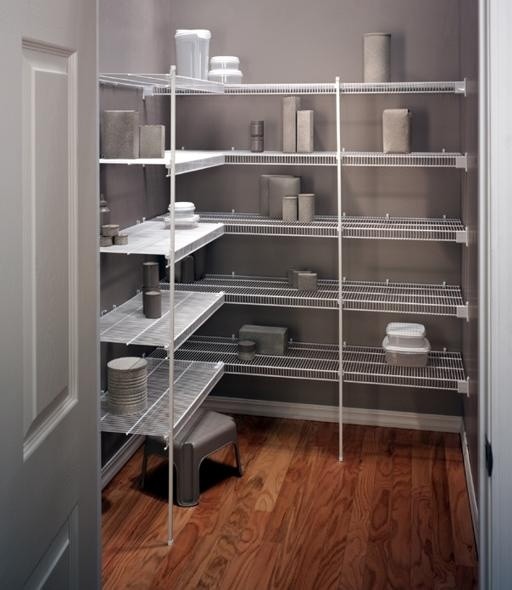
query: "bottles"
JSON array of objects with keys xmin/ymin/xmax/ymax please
[{"xmin": 364, "ymin": 32, "xmax": 391, "ymax": 83}]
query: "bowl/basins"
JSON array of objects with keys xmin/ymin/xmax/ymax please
[
  {"xmin": 208, "ymin": 70, "xmax": 243, "ymax": 87},
  {"xmin": 210, "ymin": 54, "xmax": 239, "ymax": 70}
]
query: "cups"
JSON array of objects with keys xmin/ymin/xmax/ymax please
[
  {"xmin": 250, "ymin": 120, "xmax": 265, "ymax": 151},
  {"xmin": 173, "ymin": 28, "xmax": 211, "ymax": 80},
  {"xmin": 98, "ymin": 224, "xmax": 129, "ymax": 246},
  {"xmin": 296, "ymin": 192, "xmax": 315, "ymax": 222},
  {"xmin": 237, "ymin": 339, "xmax": 256, "ymax": 362},
  {"xmin": 141, "ymin": 261, "xmax": 162, "ymax": 319},
  {"xmin": 281, "ymin": 196, "xmax": 297, "ymax": 222}
]
